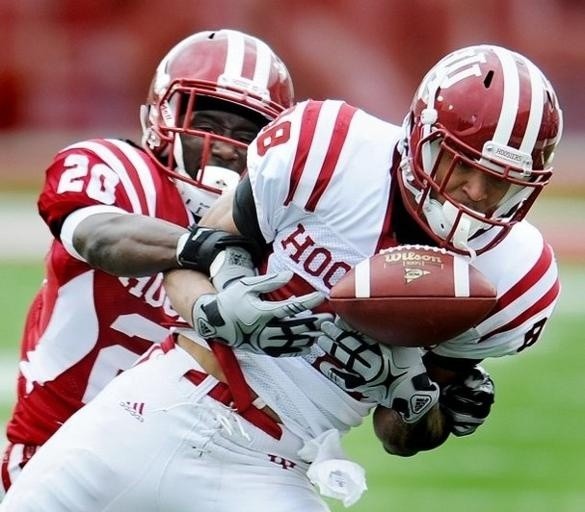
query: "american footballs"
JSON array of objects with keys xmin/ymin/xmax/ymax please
[{"xmin": 329, "ymin": 244, "xmax": 497, "ymax": 348}]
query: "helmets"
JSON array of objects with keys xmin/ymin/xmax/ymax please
[
  {"xmin": 141, "ymin": 28, "xmax": 297, "ymax": 157},
  {"xmin": 398, "ymin": 45, "xmax": 564, "ymax": 219}
]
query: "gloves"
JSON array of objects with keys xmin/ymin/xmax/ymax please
[
  {"xmin": 178, "ymin": 225, "xmax": 261, "ymax": 301},
  {"xmin": 315, "ymin": 313, "xmax": 441, "ymax": 425},
  {"xmin": 443, "ymin": 364, "xmax": 497, "ymax": 437},
  {"xmin": 190, "ymin": 271, "xmax": 333, "ymax": 355}
]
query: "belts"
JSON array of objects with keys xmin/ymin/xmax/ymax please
[{"xmin": 158, "ymin": 318, "xmax": 284, "ymax": 442}]
